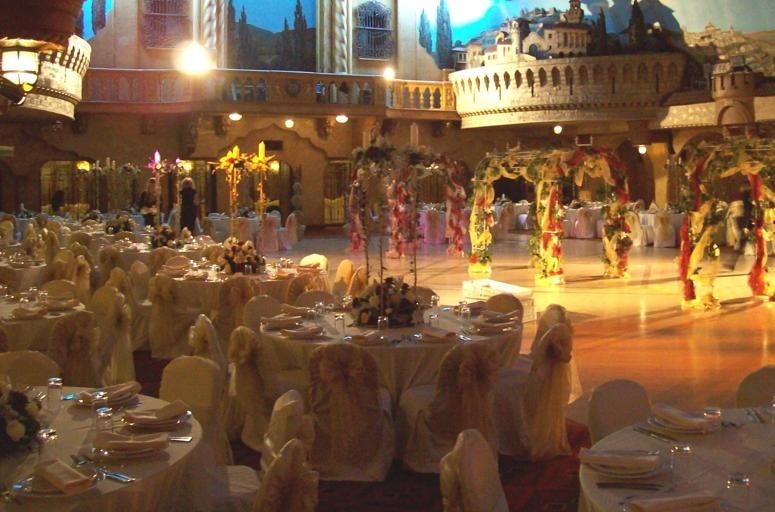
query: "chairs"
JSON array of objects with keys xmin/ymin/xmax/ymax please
[
  {"xmin": 585, "ymin": 379, "xmax": 652, "ymax": 444},
  {"xmin": 1, "ymin": 200, "xmax": 583, "ymax": 512},
  {"xmin": 736, "ymin": 365, "xmax": 775, "ymax": 407}
]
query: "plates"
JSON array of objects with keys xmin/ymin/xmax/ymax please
[
  {"xmin": 263, "ymin": 302, "xmax": 519, "ymax": 346},
  {"xmin": 587, "ymin": 401, "xmax": 775, "ymax": 512},
  {"xmin": 0, "ymin": 381, "xmax": 193, "ymax": 498}
]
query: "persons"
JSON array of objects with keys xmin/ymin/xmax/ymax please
[
  {"xmin": 178, "ymin": 177, "xmax": 199, "ymax": 236},
  {"xmin": 138, "ymin": 177, "xmax": 158, "ymax": 226}
]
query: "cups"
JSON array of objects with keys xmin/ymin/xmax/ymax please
[
  {"xmin": 0, "ymin": 283, "xmax": 48, "ymax": 313},
  {"xmin": 669, "ymin": 397, "xmax": 775, "ymax": 512},
  {"xmin": 189, "ymin": 256, "xmax": 294, "ymax": 279},
  {"xmin": 311, "ymin": 294, "xmax": 472, "ymax": 340},
  {"xmin": 36, "ymin": 377, "xmax": 116, "ymax": 478}
]
